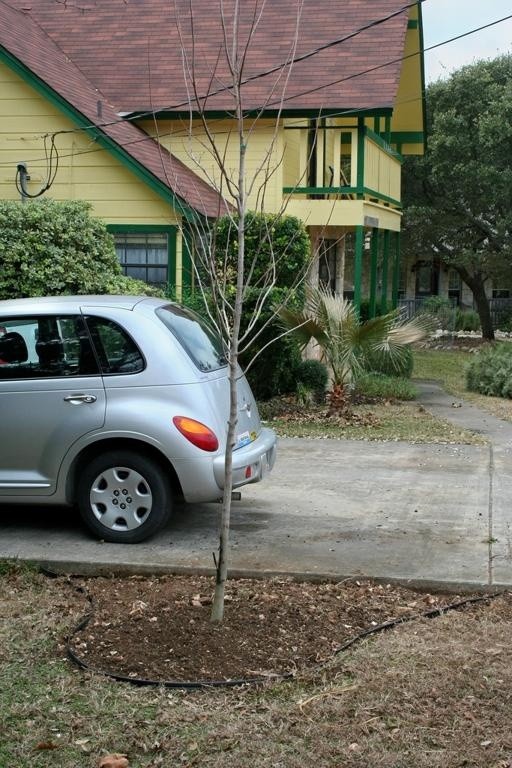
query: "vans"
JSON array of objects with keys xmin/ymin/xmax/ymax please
[{"xmin": 0, "ymin": 320, "xmax": 66, "ymax": 367}]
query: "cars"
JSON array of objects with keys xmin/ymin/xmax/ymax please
[{"xmin": 0, "ymin": 295, "xmax": 277, "ymax": 543}]
